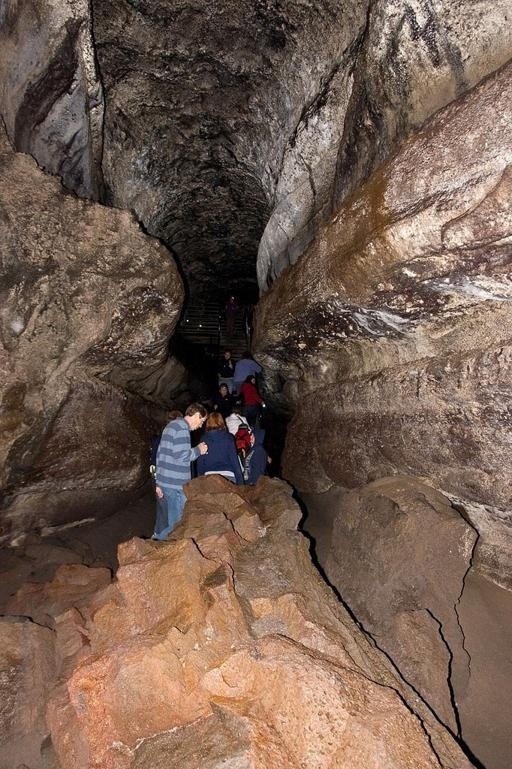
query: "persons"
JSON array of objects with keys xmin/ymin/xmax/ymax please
[
  {"xmin": 188, "ymin": 349, "xmax": 270, "ymax": 486},
  {"xmin": 150, "ymin": 403, "xmax": 209, "ymax": 543},
  {"xmin": 149, "ymin": 409, "xmax": 183, "ymax": 489}
]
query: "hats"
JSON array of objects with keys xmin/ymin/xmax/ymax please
[{"xmin": 239, "ymin": 423, "xmax": 250, "ymax": 431}]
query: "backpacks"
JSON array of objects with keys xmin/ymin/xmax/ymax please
[{"xmin": 236, "ymin": 434, "xmax": 249, "ymax": 453}]
